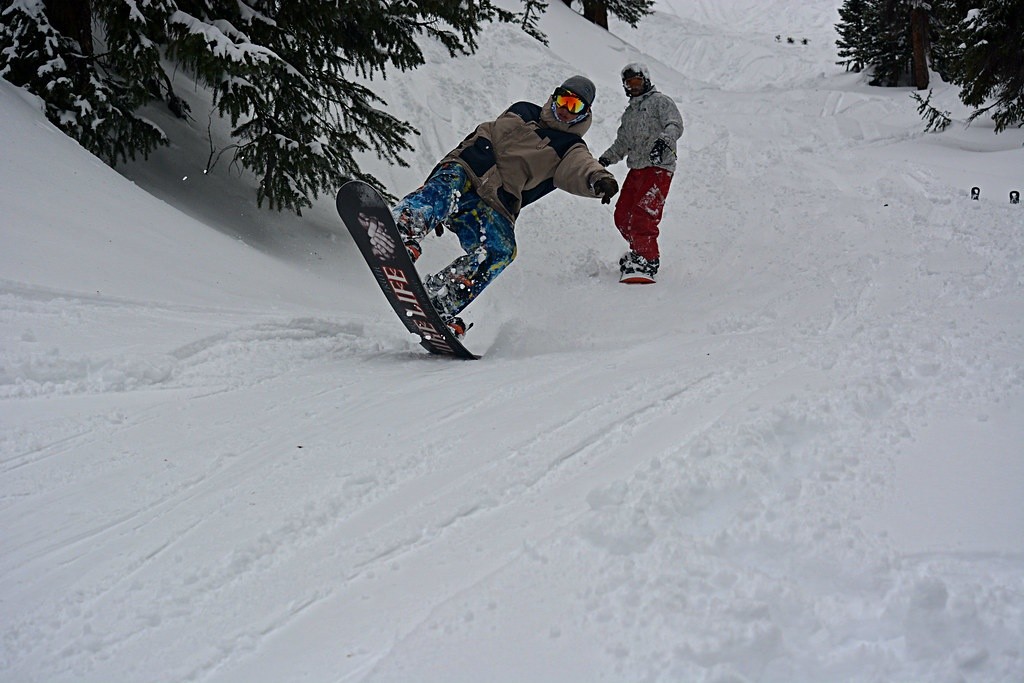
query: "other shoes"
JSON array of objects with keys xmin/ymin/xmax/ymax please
[
  {"xmin": 422, "ymin": 283, "xmax": 466, "ymax": 337},
  {"xmin": 621, "ymin": 260, "xmax": 658, "ymax": 274},
  {"xmin": 396, "ymin": 211, "xmax": 422, "ymax": 263},
  {"xmin": 619, "ymin": 251, "xmax": 637, "ymax": 264}
]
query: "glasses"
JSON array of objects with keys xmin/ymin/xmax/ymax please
[{"xmin": 552, "ymin": 87, "xmax": 588, "ymax": 115}]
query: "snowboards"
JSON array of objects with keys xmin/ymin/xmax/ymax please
[
  {"xmin": 619, "ymin": 270, "xmax": 656, "ymax": 284},
  {"xmin": 335, "ymin": 180, "xmax": 482, "ymax": 360}
]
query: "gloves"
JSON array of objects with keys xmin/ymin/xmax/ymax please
[
  {"xmin": 648, "ymin": 140, "xmax": 666, "ymax": 164},
  {"xmin": 598, "ymin": 157, "xmax": 605, "ymax": 167},
  {"xmin": 595, "ymin": 178, "xmax": 619, "ymax": 206}
]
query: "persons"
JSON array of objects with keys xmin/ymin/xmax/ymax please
[
  {"xmin": 394, "ymin": 75, "xmax": 619, "ymax": 341},
  {"xmin": 598, "ymin": 59, "xmax": 685, "ymax": 284}
]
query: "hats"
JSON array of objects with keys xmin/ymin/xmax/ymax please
[
  {"xmin": 562, "ymin": 75, "xmax": 595, "ymax": 104},
  {"xmin": 621, "ymin": 63, "xmax": 651, "ymax": 92}
]
